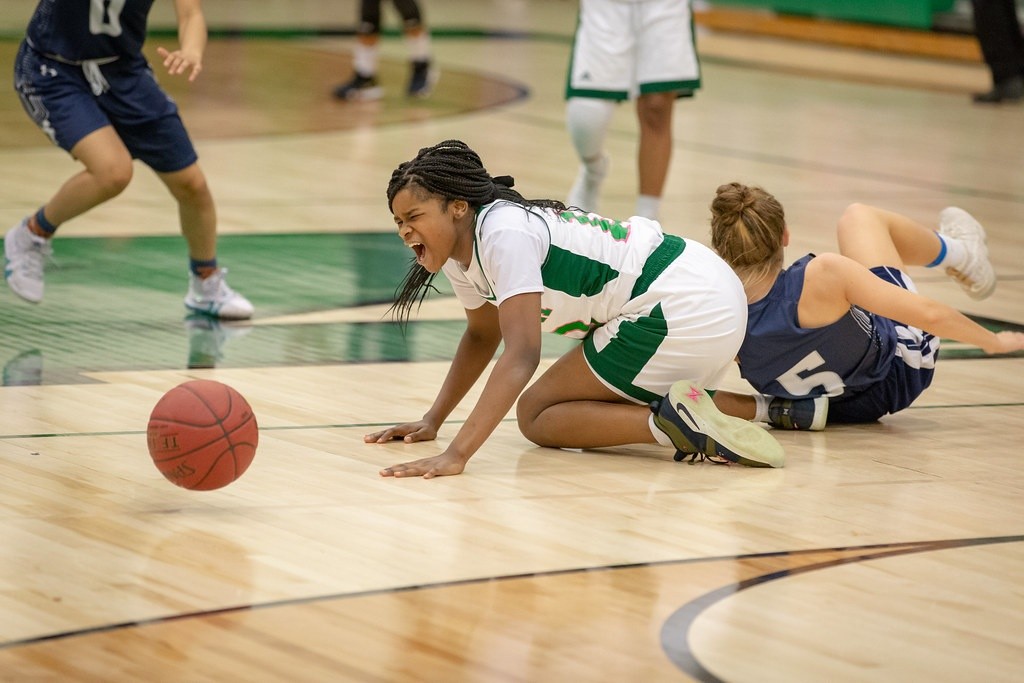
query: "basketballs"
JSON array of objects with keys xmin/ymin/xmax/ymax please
[{"xmin": 146, "ymin": 381, "xmax": 259, "ymax": 491}]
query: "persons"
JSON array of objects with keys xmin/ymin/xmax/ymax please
[
  {"xmin": 365, "ymin": 140, "xmax": 829, "ymax": 479},
  {"xmin": 565, "ymin": 0, "xmax": 701, "ymax": 221},
  {"xmin": 708, "ymin": 182, "xmax": 1024, "ymax": 425},
  {"xmin": 967, "ymin": 0, "xmax": 1024, "ymax": 104},
  {"xmin": 332, "ymin": 1, "xmax": 433, "ymax": 100},
  {"xmin": 1, "ymin": 0, "xmax": 255, "ymax": 319}
]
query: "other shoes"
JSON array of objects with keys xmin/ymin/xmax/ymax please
[
  {"xmin": 408, "ymin": 58, "xmax": 440, "ymax": 99},
  {"xmin": 970, "ymin": 82, "xmax": 1024, "ymax": 102},
  {"xmin": 570, "ymin": 157, "xmax": 609, "ymax": 214},
  {"xmin": 333, "ymin": 74, "xmax": 385, "ymax": 102}
]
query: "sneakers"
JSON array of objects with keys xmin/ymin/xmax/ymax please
[
  {"xmin": 650, "ymin": 379, "xmax": 784, "ymax": 468},
  {"xmin": 5, "ymin": 216, "xmax": 58, "ymax": 304},
  {"xmin": 938, "ymin": 207, "xmax": 996, "ymax": 301},
  {"xmin": 184, "ymin": 267, "xmax": 254, "ymax": 320},
  {"xmin": 768, "ymin": 397, "xmax": 828, "ymax": 431}
]
